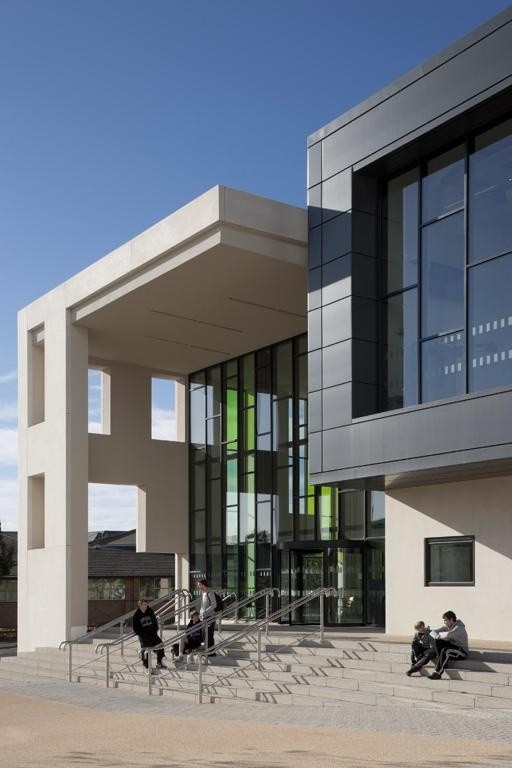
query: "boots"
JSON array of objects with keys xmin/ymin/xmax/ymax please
[{"xmin": 406, "ymin": 668, "xmax": 414, "ymax": 677}]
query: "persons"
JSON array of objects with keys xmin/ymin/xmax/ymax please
[
  {"xmin": 170, "ymin": 611, "xmax": 204, "ymax": 661},
  {"xmin": 426, "ymin": 610, "xmax": 470, "ymax": 679},
  {"xmin": 197, "ymin": 577, "xmax": 224, "ymax": 658},
  {"xmin": 131, "ymin": 598, "xmax": 168, "ymax": 673},
  {"xmin": 406, "ymin": 621, "xmax": 438, "ymax": 676}
]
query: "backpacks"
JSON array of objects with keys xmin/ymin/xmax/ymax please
[{"xmin": 206, "ymin": 591, "xmax": 224, "ymax": 613}]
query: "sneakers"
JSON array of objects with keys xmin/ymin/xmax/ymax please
[
  {"xmin": 173, "ymin": 655, "xmax": 184, "ymax": 663},
  {"xmin": 443, "ymin": 663, "xmax": 449, "ymax": 669},
  {"xmin": 156, "ymin": 663, "xmax": 167, "ymax": 669},
  {"xmin": 145, "ymin": 668, "xmax": 149, "ymax": 676},
  {"xmin": 202, "ymin": 653, "xmax": 217, "ymax": 657},
  {"xmin": 427, "ymin": 671, "xmax": 442, "ymax": 680}
]
